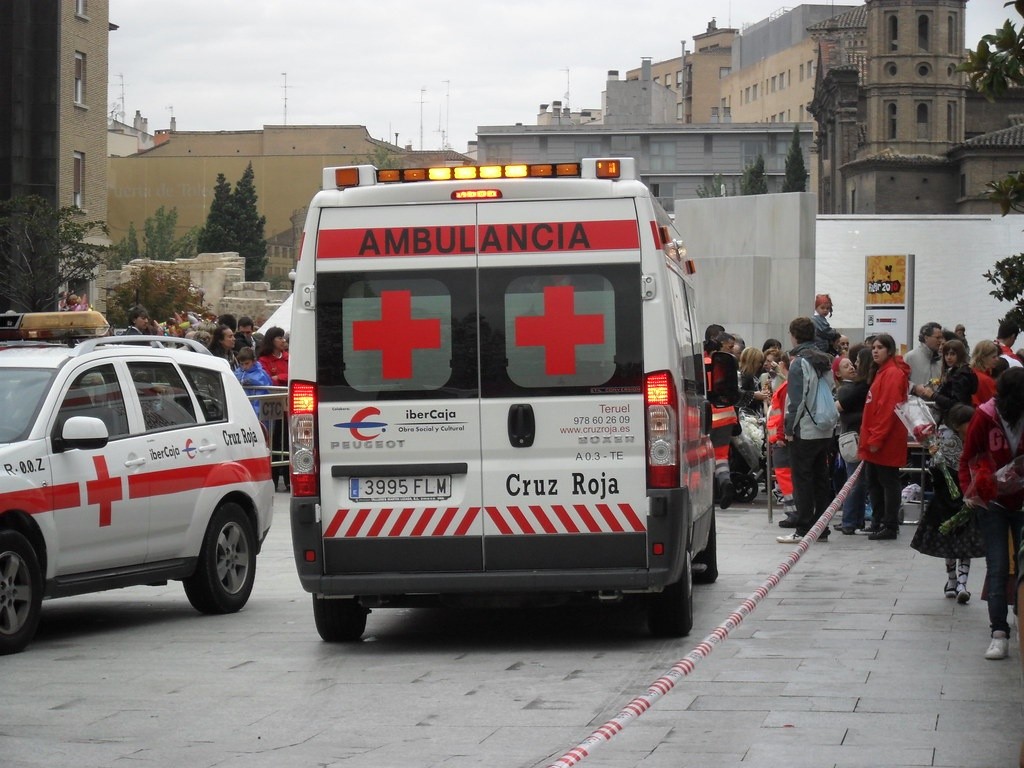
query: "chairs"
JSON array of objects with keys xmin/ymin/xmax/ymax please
[{"xmin": 82, "ymin": 375, "xmax": 121, "ymax": 436}]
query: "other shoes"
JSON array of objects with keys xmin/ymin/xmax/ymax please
[
  {"xmin": 945, "ymin": 582, "xmax": 957, "ymax": 597},
  {"xmin": 780, "ymin": 512, "xmax": 799, "ymax": 527},
  {"xmin": 957, "ymin": 590, "xmax": 971, "ymax": 603},
  {"xmin": 833, "ymin": 522, "xmax": 900, "ymax": 539},
  {"xmin": 720, "ymin": 481, "xmax": 735, "ymax": 509}
]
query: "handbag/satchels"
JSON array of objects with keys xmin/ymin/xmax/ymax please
[{"xmin": 838, "ymin": 430, "xmax": 863, "ymax": 463}]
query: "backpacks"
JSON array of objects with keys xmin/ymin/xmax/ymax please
[{"xmin": 804, "ymin": 366, "xmax": 841, "ymax": 430}]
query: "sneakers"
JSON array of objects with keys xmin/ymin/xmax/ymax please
[
  {"xmin": 985, "ymin": 630, "xmax": 1010, "ymax": 659},
  {"xmin": 816, "ymin": 537, "xmax": 828, "ymax": 542},
  {"xmin": 777, "ymin": 532, "xmax": 803, "ymax": 543}
]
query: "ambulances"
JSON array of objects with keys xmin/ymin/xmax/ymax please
[
  {"xmin": 0, "ymin": 309, "xmax": 275, "ymax": 656},
  {"xmin": 288, "ymin": 157, "xmax": 718, "ymax": 642}
]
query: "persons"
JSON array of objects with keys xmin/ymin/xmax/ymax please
[
  {"xmin": 119, "ymin": 303, "xmax": 293, "ymax": 493},
  {"xmin": 57, "ymin": 291, "xmax": 89, "ymax": 311},
  {"xmin": 704, "ymin": 292, "xmax": 1024, "ymax": 659}
]
